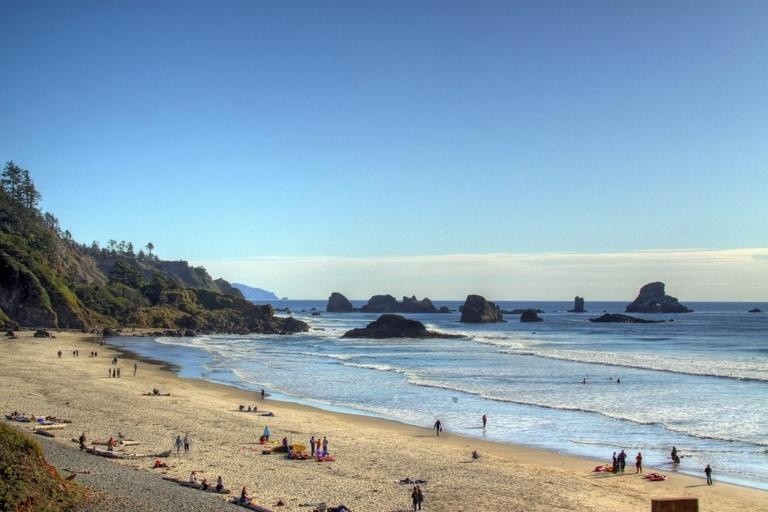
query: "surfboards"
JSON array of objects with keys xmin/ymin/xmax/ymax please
[{"xmin": 272, "ymin": 444, "xmax": 305, "ymax": 452}]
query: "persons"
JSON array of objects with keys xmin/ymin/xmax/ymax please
[
  {"xmin": 411, "ymin": 487, "xmax": 418, "ymax": 511},
  {"xmin": 261, "ymin": 388, "xmax": 265, "ymax": 399},
  {"xmin": 190, "ymin": 472, "xmax": 195, "ymax": 482},
  {"xmin": 133, "ymin": 363, "xmax": 137, "ymax": 375},
  {"xmin": 593, "ymin": 463, "xmax": 609, "ymax": 471},
  {"xmin": 613, "ymin": 451, "xmax": 618, "ymax": 473},
  {"xmin": 176, "ymin": 435, "xmax": 189, "ymax": 452},
  {"xmin": 242, "ymin": 487, "xmax": 246, "ymax": 498},
  {"xmin": 635, "ymin": 452, "xmax": 642, "ymax": 473},
  {"xmin": 218, "ymin": 476, "xmax": 222, "ymax": 487},
  {"xmin": 109, "ymin": 358, "xmax": 120, "ymax": 377},
  {"xmin": 108, "ymin": 437, "xmax": 114, "ymax": 451},
  {"xmin": 58, "ymin": 351, "xmax": 97, "ymax": 357},
  {"xmin": 482, "ymin": 413, "xmax": 488, "ymax": 429},
  {"xmin": 433, "ymin": 420, "xmax": 441, "ymax": 437},
  {"xmin": 704, "ymin": 463, "xmax": 712, "ymax": 484},
  {"xmin": 260, "ymin": 426, "xmax": 270, "ymax": 442},
  {"xmin": 79, "ymin": 433, "xmax": 86, "ymax": 450},
  {"xmin": 618, "ymin": 449, "xmax": 627, "ymax": 475},
  {"xmin": 202, "ymin": 479, "xmax": 206, "ymax": 485},
  {"xmin": 416, "ymin": 485, "xmax": 424, "ymax": 510},
  {"xmin": 153, "ymin": 388, "xmax": 159, "ymax": 395},
  {"xmin": 671, "ymin": 446, "xmax": 679, "ymax": 464},
  {"xmin": 240, "ymin": 405, "xmax": 257, "ymax": 411},
  {"xmin": 310, "ymin": 436, "xmax": 328, "ymax": 455},
  {"xmin": 643, "ymin": 471, "xmax": 666, "ymax": 481}
]
